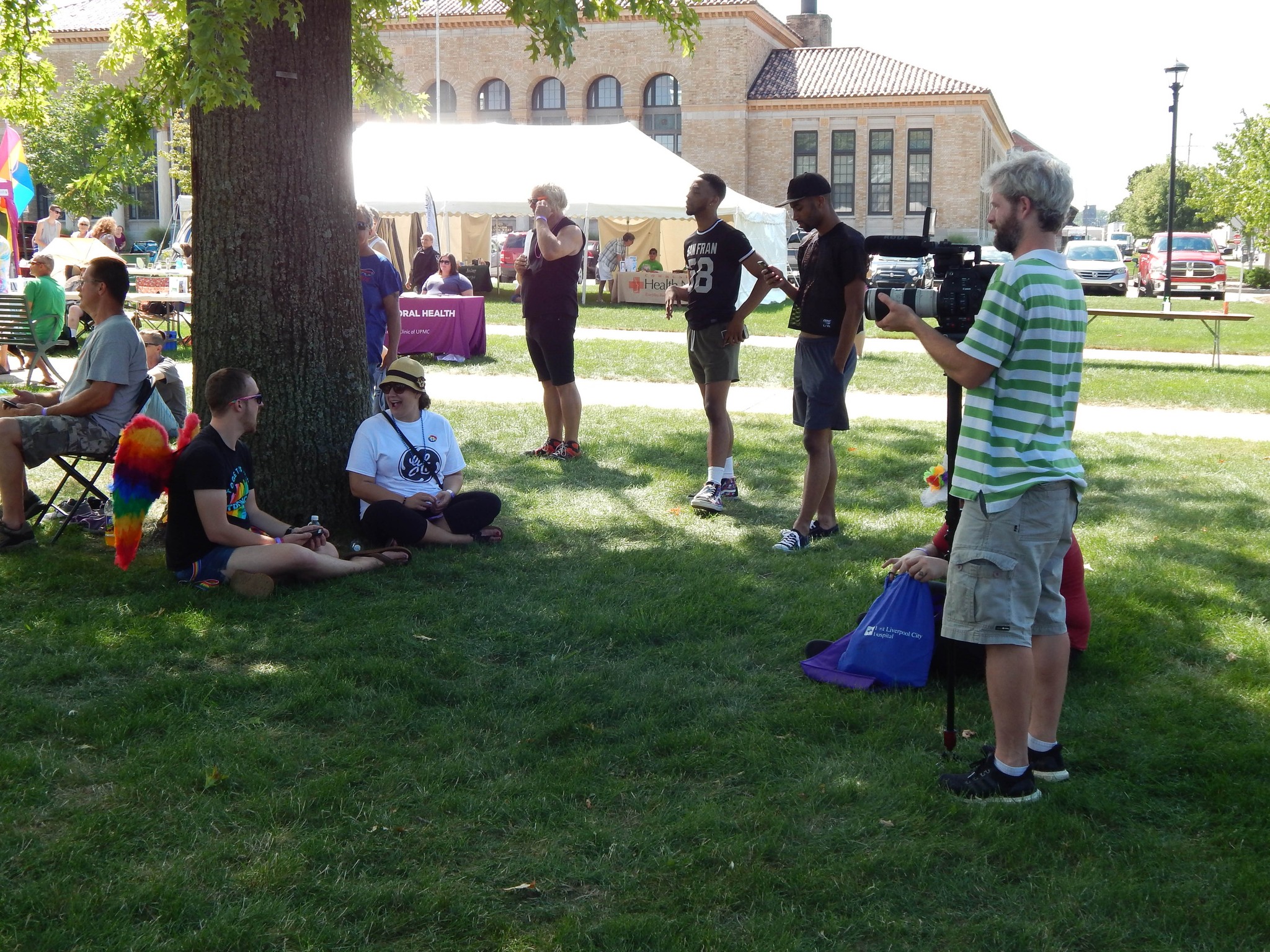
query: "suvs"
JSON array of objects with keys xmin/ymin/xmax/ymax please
[
  {"xmin": 1250, "ymin": 245, "xmax": 1259, "ymax": 260},
  {"xmin": 1138, "ymin": 232, "xmax": 1233, "ymax": 301},
  {"xmin": 871, "ymin": 254, "xmax": 935, "ymax": 290},
  {"xmin": 787, "ymin": 227, "xmax": 810, "ymax": 287},
  {"xmin": 500, "ymin": 231, "xmax": 528, "ymax": 283}
]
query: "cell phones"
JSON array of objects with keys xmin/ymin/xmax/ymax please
[
  {"xmin": 1, "ymin": 398, "xmax": 19, "ymax": 409},
  {"xmin": 757, "ymin": 261, "xmax": 780, "ymax": 281},
  {"xmin": 292, "ymin": 528, "xmax": 323, "ymax": 537},
  {"xmin": 721, "ymin": 324, "xmax": 749, "ymax": 345}
]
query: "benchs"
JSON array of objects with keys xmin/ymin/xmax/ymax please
[{"xmin": 0, "ymin": 293, "xmax": 70, "ymax": 390}]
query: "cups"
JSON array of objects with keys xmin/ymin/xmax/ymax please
[
  {"xmin": 156, "ymin": 262, "xmax": 161, "ymax": 271},
  {"xmin": 176, "ymin": 257, "xmax": 183, "ymax": 269}
]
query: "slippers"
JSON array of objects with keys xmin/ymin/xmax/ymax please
[
  {"xmin": 230, "ymin": 569, "xmax": 274, "ymax": 597},
  {"xmin": 0, "ymin": 362, "xmax": 57, "ymax": 387},
  {"xmin": 470, "ymin": 525, "xmax": 503, "ymax": 544},
  {"xmin": 387, "ymin": 537, "xmax": 399, "ymax": 546},
  {"xmin": 342, "ymin": 546, "xmax": 413, "ymax": 567}
]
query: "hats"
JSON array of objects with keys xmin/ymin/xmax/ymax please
[
  {"xmin": 379, "ymin": 356, "xmax": 426, "ymax": 392},
  {"xmin": 773, "ymin": 172, "xmax": 831, "ymax": 207}
]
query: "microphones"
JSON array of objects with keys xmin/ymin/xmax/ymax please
[{"xmin": 864, "ymin": 236, "xmax": 941, "ymax": 258}]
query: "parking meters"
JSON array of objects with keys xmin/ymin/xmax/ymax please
[{"xmin": 1238, "ymin": 244, "xmax": 1248, "ymax": 302}]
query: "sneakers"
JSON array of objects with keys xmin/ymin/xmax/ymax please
[
  {"xmin": 935, "ymin": 751, "xmax": 1042, "ymax": 803},
  {"xmin": 1025, "ymin": 742, "xmax": 1069, "ymax": 783},
  {"xmin": 808, "ymin": 520, "xmax": 839, "ymax": 541},
  {"xmin": 525, "ymin": 438, "xmax": 563, "ymax": 457},
  {"xmin": 0, "ymin": 488, "xmax": 113, "ymax": 555},
  {"xmin": 691, "ymin": 481, "xmax": 724, "ymax": 511},
  {"xmin": 687, "ymin": 476, "xmax": 738, "ymax": 499},
  {"xmin": 549, "ymin": 441, "xmax": 581, "ymax": 460},
  {"xmin": 771, "ymin": 528, "xmax": 810, "ymax": 553}
]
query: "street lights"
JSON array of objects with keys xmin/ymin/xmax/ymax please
[{"xmin": 1159, "ymin": 58, "xmax": 1190, "ymax": 321}]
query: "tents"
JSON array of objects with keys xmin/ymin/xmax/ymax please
[{"xmin": 352, "ymin": 121, "xmax": 788, "ymax": 311}]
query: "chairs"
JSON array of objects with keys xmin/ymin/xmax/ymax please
[
  {"xmin": 66, "ymin": 264, "xmax": 95, "ymax": 339},
  {"xmin": 134, "ymin": 275, "xmax": 176, "ymax": 332},
  {"xmin": 484, "ymin": 261, "xmax": 490, "ymax": 267},
  {"xmin": 1194, "ymin": 240, "xmax": 1206, "ymax": 250},
  {"xmin": 29, "ymin": 372, "xmax": 157, "ymax": 547}
]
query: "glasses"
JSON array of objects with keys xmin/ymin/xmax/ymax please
[
  {"xmin": 649, "ymin": 252, "xmax": 656, "ymax": 255},
  {"xmin": 78, "ymin": 279, "xmax": 105, "ymax": 287},
  {"xmin": 528, "ymin": 196, "xmax": 548, "ymax": 205},
  {"xmin": 79, "ymin": 223, "xmax": 88, "ymax": 227},
  {"xmin": 52, "ymin": 209, "xmax": 61, "ymax": 215},
  {"xmin": 438, "ymin": 260, "xmax": 451, "ymax": 265},
  {"xmin": 357, "ymin": 221, "xmax": 371, "ymax": 230},
  {"xmin": 31, "ymin": 261, "xmax": 46, "ymax": 265},
  {"xmin": 228, "ymin": 392, "xmax": 263, "ymax": 405},
  {"xmin": 381, "ymin": 384, "xmax": 415, "ymax": 394},
  {"xmin": 144, "ymin": 342, "xmax": 159, "ymax": 347}
]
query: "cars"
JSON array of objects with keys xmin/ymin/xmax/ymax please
[
  {"xmin": 1063, "ymin": 240, "xmax": 1132, "ymax": 297},
  {"xmin": 581, "ymin": 240, "xmax": 600, "ymax": 278},
  {"xmin": 964, "ymin": 246, "xmax": 1014, "ymax": 266},
  {"xmin": 1132, "ymin": 258, "xmax": 1139, "ymax": 287},
  {"xmin": 491, "ymin": 234, "xmax": 508, "ymax": 267}
]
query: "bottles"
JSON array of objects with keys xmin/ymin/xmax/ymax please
[
  {"xmin": 308, "ymin": 515, "xmax": 322, "ymax": 526},
  {"xmin": 10, "ymin": 263, "xmax": 14, "ymax": 278},
  {"xmin": 104, "ymin": 492, "xmax": 116, "ymax": 547},
  {"xmin": 350, "ymin": 539, "xmax": 364, "ymax": 552}
]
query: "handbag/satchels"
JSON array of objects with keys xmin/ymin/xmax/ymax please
[{"xmin": 837, "ymin": 572, "xmax": 935, "ymax": 689}]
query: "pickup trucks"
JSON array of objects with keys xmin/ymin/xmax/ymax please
[{"xmin": 18, "ymin": 221, "xmax": 71, "ymax": 260}]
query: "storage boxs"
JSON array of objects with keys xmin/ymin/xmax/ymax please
[{"xmin": 162, "ymin": 331, "xmax": 177, "ymax": 350}]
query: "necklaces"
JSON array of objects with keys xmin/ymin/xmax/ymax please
[
  {"xmin": 535, "ymin": 216, "xmax": 565, "ymax": 258},
  {"xmin": 391, "ymin": 412, "xmax": 426, "ymax": 467},
  {"xmin": 368, "ymin": 234, "xmax": 378, "ymax": 240}
]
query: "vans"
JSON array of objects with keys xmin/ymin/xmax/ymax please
[{"xmin": 1107, "ymin": 232, "xmax": 1133, "ymax": 255}]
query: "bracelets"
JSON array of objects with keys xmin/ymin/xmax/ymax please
[
  {"xmin": 280, "ymin": 537, "xmax": 285, "ymax": 543},
  {"xmin": 402, "ymin": 496, "xmax": 407, "ymax": 506},
  {"xmin": 284, "ymin": 525, "xmax": 296, "ymax": 536},
  {"xmin": 910, "ymin": 547, "xmax": 930, "ymax": 556},
  {"xmin": 42, "ymin": 407, "xmax": 48, "ymax": 416},
  {"xmin": 274, "ymin": 537, "xmax": 282, "ymax": 544},
  {"xmin": 536, "ymin": 216, "xmax": 547, "ymax": 222},
  {"xmin": 445, "ymin": 489, "xmax": 455, "ymax": 498}
]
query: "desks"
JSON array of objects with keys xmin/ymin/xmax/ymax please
[
  {"xmin": 382, "ymin": 292, "xmax": 487, "ymax": 363},
  {"xmin": 459, "ymin": 265, "xmax": 493, "ymax": 292},
  {"xmin": 65, "ymin": 268, "xmax": 192, "ymax": 350},
  {"xmin": 610, "ymin": 270, "xmax": 689, "ymax": 305}
]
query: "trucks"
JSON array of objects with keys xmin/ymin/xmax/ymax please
[{"xmin": 1107, "ymin": 222, "xmax": 1128, "ymax": 232}]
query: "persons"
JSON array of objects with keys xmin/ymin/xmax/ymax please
[
  {"xmin": 876, "ymin": 152, "xmax": 1088, "ymax": 803},
  {"xmin": 0, "ymin": 183, "xmax": 663, "ymax": 598},
  {"xmin": 665, "ymin": 174, "xmax": 773, "ymax": 512},
  {"xmin": 853, "ymin": 282, "xmax": 868, "ymax": 359},
  {"xmin": 881, "ymin": 496, "xmax": 1092, "ymax": 681},
  {"xmin": 762, "ymin": 172, "xmax": 869, "ymax": 552}
]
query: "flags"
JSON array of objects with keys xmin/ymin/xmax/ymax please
[{"xmin": 424, "ymin": 186, "xmax": 440, "ymax": 253}]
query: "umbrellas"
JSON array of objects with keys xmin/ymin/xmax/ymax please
[{"xmin": 33, "ymin": 237, "xmax": 127, "ymax": 286}]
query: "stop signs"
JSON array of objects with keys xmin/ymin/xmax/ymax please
[{"xmin": 1233, "ymin": 235, "xmax": 1241, "ymax": 245}]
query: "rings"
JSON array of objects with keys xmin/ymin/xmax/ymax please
[{"xmin": 918, "ymin": 570, "xmax": 925, "ymax": 577}]
